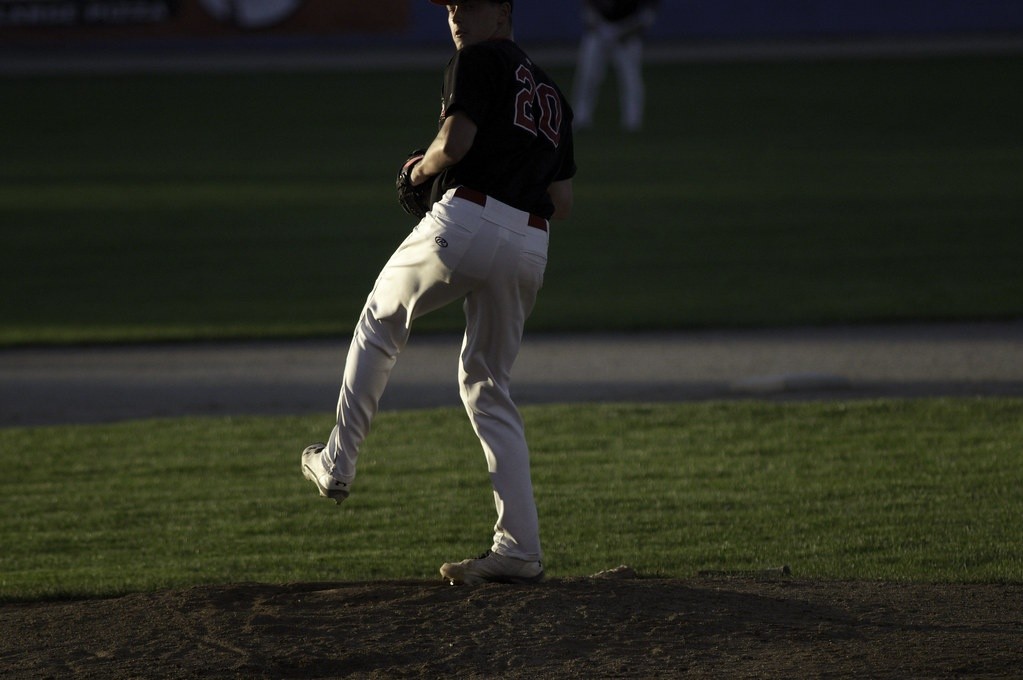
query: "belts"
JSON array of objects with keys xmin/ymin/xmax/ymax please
[{"xmin": 455, "ymin": 188, "xmax": 547, "ymax": 232}]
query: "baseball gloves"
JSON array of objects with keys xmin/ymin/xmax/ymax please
[{"xmin": 396, "ymin": 148, "xmax": 451, "ymax": 220}]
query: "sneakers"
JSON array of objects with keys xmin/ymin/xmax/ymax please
[
  {"xmin": 438, "ymin": 549, "xmax": 545, "ymax": 587},
  {"xmin": 301, "ymin": 442, "xmax": 352, "ymax": 505}
]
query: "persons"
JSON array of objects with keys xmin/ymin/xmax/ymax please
[
  {"xmin": 573, "ymin": 0, "xmax": 659, "ymax": 132},
  {"xmin": 300, "ymin": 0, "xmax": 577, "ymax": 583}
]
